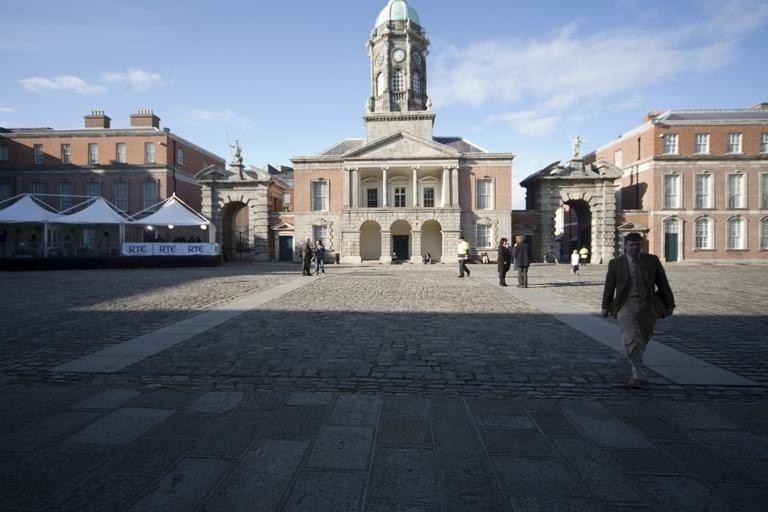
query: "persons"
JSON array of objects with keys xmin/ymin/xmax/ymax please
[
  {"xmin": 455, "ymin": 236, "xmax": 471, "ymax": 278},
  {"xmin": 569, "ymin": 248, "xmax": 580, "ymax": 275},
  {"xmin": 144, "ymin": 231, "xmax": 202, "ymax": 243},
  {"xmin": 512, "ymin": 235, "xmax": 529, "ymax": 289},
  {"xmin": 599, "ymin": 232, "xmax": 676, "ymax": 388},
  {"xmin": 513, "ymin": 244, "xmax": 517, "ymax": 263},
  {"xmin": 579, "ymin": 245, "xmax": 589, "ymax": 266},
  {"xmin": 572, "ymin": 135, "xmax": 581, "ymax": 159},
  {"xmin": 311, "ymin": 239, "xmax": 325, "ymax": 276},
  {"xmin": 481, "ymin": 251, "xmax": 488, "ymax": 264},
  {"xmin": 229, "ymin": 140, "xmax": 244, "ymax": 164},
  {"xmin": 506, "ymin": 242, "xmax": 513, "ymax": 263},
  {"xmin": 391, "ymin": 252, "xmax": 402, "ymax": 264},
  {"xmin": 424, "ymin": 253, "xmax": 432, "ymax": 265},
  {"xmin": 498, "ymin": 238, "xmax": 511, "ymax": 287},
  {"xmin": 299, "ymin": 238, "xmax": 314, "ymax": 277}
]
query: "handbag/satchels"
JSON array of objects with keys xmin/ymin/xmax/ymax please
[{"xmin": 647, "ymin": 290, "xmax": 669, "ymax": 320}]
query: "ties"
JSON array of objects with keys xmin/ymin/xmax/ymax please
[{"xmin": 635, "ymin": 260, "xmax": 648, "ymax": 302}]
